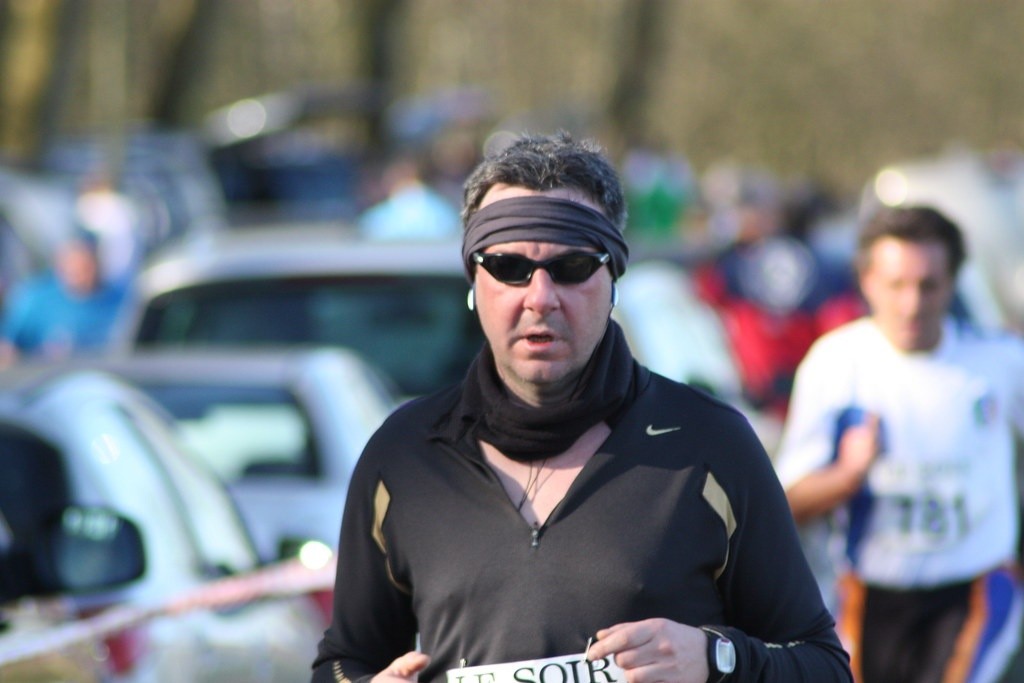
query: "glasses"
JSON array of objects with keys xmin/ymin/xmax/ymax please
[{"xmin": 473, "ymin": 250, "xmax": 609, "ymax": 286}]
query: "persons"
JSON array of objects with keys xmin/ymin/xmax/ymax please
[
  {"xmin": 311, "ymin": 128, "xmax": 850, "ymax": 683},
  {"xmin": 355, "ymin": 153, "xmax": 460, "ymax": 245},
  {"xmin": 770, "ymin": 206, "xmax": 1024, "ymax": 683},
  {"xmin": 0, "ymin": 225, "xmax": 124, "ymax": 369}
]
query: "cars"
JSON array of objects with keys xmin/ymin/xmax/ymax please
[{"xmin": 0, "ymin": 148, "xmax": 1024, "ymax": 683}]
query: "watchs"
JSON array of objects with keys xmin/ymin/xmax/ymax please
[{"xmin": 701, "ymin": 627, "xmax": 736, "ymax": 683}]
